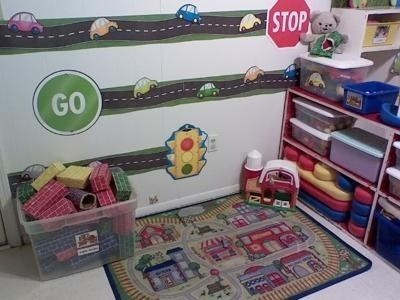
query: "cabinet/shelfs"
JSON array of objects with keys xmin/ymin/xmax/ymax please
[{"xmin": 279, "ymin": 6, "xmax": 400, "ymax": 274}]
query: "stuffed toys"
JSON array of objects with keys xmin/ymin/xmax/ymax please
[{"xmin": 298, "ymin": 10, "xmax": 349, "ymax": 59}]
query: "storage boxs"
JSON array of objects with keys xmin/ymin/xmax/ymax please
[{"xmin": 16, "ymin": 185, "xmax": 137, "ymax": 281}]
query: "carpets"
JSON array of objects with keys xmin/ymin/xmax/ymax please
[{"xmin": 104, "ymin": 193, "xmax": 373, "ymax": 300}]
